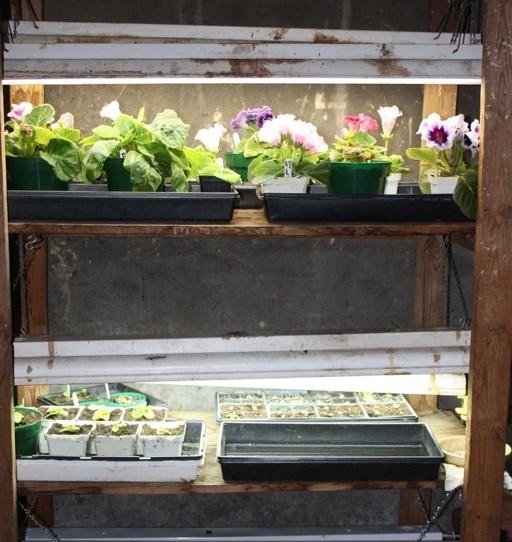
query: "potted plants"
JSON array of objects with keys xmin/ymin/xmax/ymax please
[
  {"xmin": 91, "ymin": 420, "xmax": 140, "ymax": 457},
  {"xmin": 76, "ymin": 409, "xmax": 125, "ymax": 454},
  {"xmin": 218, "ymin": 392, "xmax": 416, "ymax": 423},
  {"xmin": 45, "ymin": 422, "xmax": 93, "ymax": 457},
  {"xmin": 136, "ymin": 422, "xmax": 186, "ymax": 458},
  {"xmin": 44, "ymin": 386, "xmax": 151, "ymax": 408},
  {"xmin": 121, "ymin": 409, "xmax": 165, "ymax": 453},
  {"xmin": 38, "ymin": 408, "xmax": 80, "ymax": 451},
  {"xmin": 15, "ymin": 407, "xmax": 42, "ymax": 454}
]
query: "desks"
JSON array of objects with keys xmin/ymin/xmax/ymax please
[
  {"xmin": 4, "ymin": 206, "xmax": 475, "ymax": 342},
  {"xmin": 14, "ymin": 409, "xmax": 447, "ymax": 532}
]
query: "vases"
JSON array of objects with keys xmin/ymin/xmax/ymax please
[
  {"xmin": 383, "ymin": 175, "xmax": 401, "ymax": 194},
  {"xmin": 423, "ymin": 173, "xmax": 468, "ymax": 195},
  {"xmin": 197, "ymin": 172, "xmax": 233, "ymax": 192},
  {"xmin": 224, "ymin": 150, "xmax": 256, "ymax": 183},
  {"xmin": 7, "ymin": 149, "xmax": 70, "ymax": 192},
  {"xmin": 324, "ymin": 158, "xmax": 388, "ymax": 195},
  {"xmin": 253, "ymin": 174, "xmax": 311, "ymax": 195},
  {"xmin": 98, "ymin": 154, "xmax": 164, "ymax": 190}
]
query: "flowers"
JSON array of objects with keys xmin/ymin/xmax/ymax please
[
  {"xmin": 341, "ymin": 101, "xmax": 403, "ymax": 148},
  {"xmin": 96, "ymin": 101, "xmax": 130, "ymax": 127},
  {"xmin": 11, "ymin": 103, "xmax": 82, "ymax": 134},
  {"xmin": 195, "ymin": 123, "xmax": 227, "ymax": 155},
  {"xmin": 418, "ymin": 113, "xmax": 480, "ymax": 156},
  {"xmin": 232, "ymin": 107, "xmax": 331, "ymax": 157}
]
